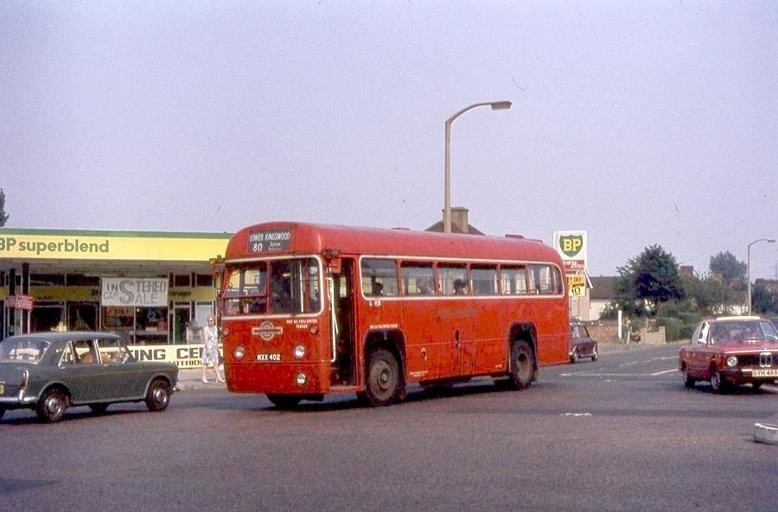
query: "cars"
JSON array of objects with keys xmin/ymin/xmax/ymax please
[
  {"xmin": 676, "ymin": 314, "xmax": 778, "ymax": 392},
  {"xmin": 566, "ymin": 321, "xmax": 599, "ymax": 362},
  {"xmin": 769, "ymin": 317, "xmax": 778, "ymax": 331},
  {"xmin": 0, "ymin": 331, "xmax": 181, "ymax": 421}
]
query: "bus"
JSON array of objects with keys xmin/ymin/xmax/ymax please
[{"xmin": 211, "ymin": 219, "xmax": 566, "ymax": 409}]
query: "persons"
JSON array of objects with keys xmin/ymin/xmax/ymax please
[
  {"xmin": 430, "ymin": 279, "xmax": 440, "ymax": 290},
  {"xmin": 200, "ymin": 315, "xmax": 225, "ymax": 384},
  {"xmin": 372, "ymin": 281, "xmax": 384, "ymax": 296},
  {"xmin": 622, "ymin": 315, "xmax": 633, "ymax": 346},
  {"xmin": 452, "ymin": 278, "xmax": 467, "ymax": 296},
  {"xmin": 87, "ymin": 340, "xmax": 117, "ymax": 364},
  {"xmin": 416, "ymin": 278, "xmax": 433, "ymax": 296}
]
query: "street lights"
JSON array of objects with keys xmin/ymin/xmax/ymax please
[
  {"xmin": 441, "ymin": 99, "xmax": 514, "ymax": 234},
  {"xmin": 748, "ymin": 238, "xmax": 776, "ymax": 315}
]
